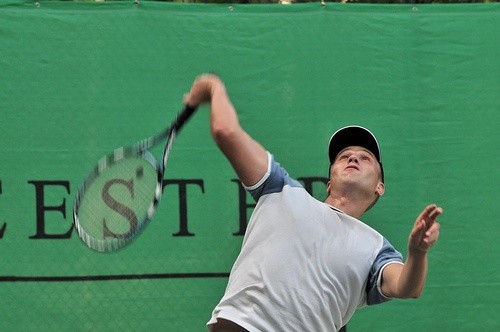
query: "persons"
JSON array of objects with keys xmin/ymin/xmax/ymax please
[{"xmin": 184, "ymin": 72, "xmax": 443, "ymax": 332}]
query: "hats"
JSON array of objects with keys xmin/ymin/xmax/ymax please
[{"xmin": 328, "ymin": 125, "xmax": 384, "ymax": 182}]
